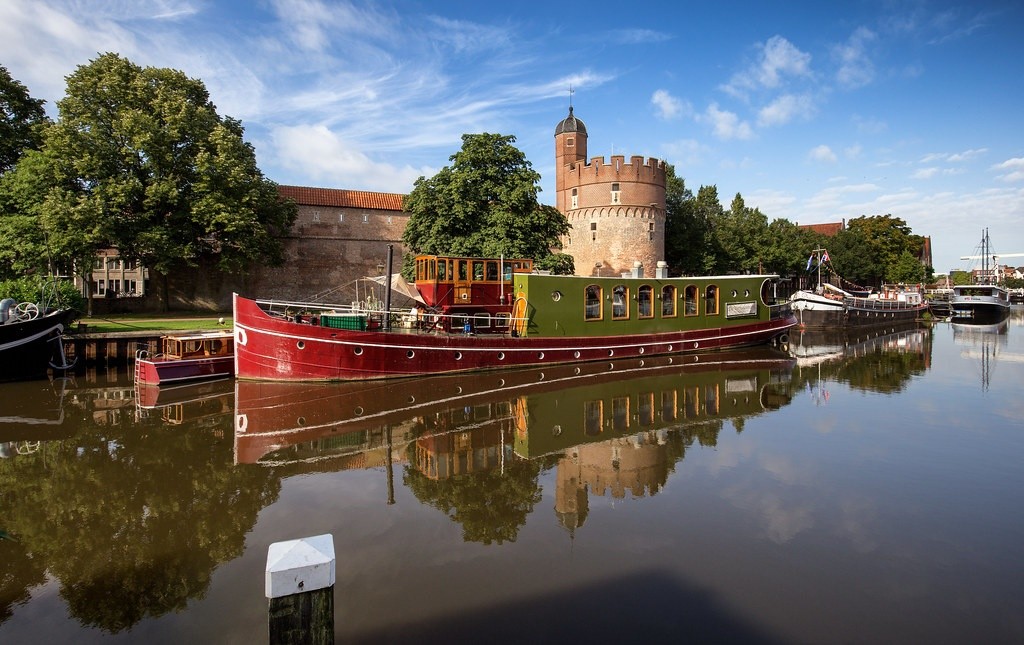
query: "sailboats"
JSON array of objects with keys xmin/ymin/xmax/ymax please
[
  {"xmin": 948, "ymin": 309, "xmax": 1013, "ymax": 392},
  {"xmin": 947, "ymin": 227, "xmax": 1012, "ymax": 312}
]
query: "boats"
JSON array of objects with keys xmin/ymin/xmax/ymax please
[
  {"xmin": 231, "ymin": 246, "xmax": 802, "ymax": 385},
  {"xmin": 785, "ymin": 243, "xmax": 932, "ymax": 333},
  {"xmin": 134, "ymin": 327, "xmax": 235, "ymax": 385},
  {"xmin": 134, "ymin": 377, "xmax": 236, "ymax": 426},
  {"xmin": 779, "ymin": 320, "xmax": 929, "ymax": 367},
  {"xmin": 0, "ymin": 374, "xmax": 69, "ymax": 460},
  {"xmin": 0, "ymin": 234, "xmax": 82, "ymax": 382},
  {"xmin": 229, "ymin": 340, "xmax": 817, "ymax": 509}
]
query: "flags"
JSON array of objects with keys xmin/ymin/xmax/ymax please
[{"xmin": 806, "ymin": 255, "xmax": 812, "ymax": 271}]
